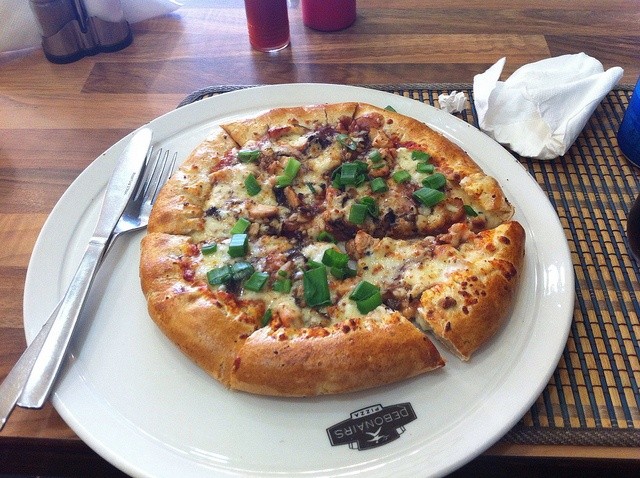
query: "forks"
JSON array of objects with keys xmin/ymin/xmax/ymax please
[{"xmin": 0, "ymin": 145, "xmax": 178, "ymax": 433}]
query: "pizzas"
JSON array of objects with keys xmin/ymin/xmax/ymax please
[{"xmin": 139, "ymin": 101, "xmax": 526, "ymax": 397}]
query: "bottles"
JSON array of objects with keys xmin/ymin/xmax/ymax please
[
  {"xmin": 244, "ymin": 0, "xmax": 291, "ymax": 53},
  {"xmin": 617, "ymin": 74, "xmax": 640, "ymax": 166},
  {"xmin": 299, "ymin": 0, "xmax": 358, "ymax": 31},
  {"xmin": 625, "ymin": 191, "xmax": 639, "ymax": 260}
]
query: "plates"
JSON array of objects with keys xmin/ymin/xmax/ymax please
[{"xmin": 21, "ymin": 79, "xmax": 576, "ymax": 476}]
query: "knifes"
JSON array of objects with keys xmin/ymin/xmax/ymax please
[{"xmin": 16, "ymin": 125, "xmax": 154, "ymax": 412}]
